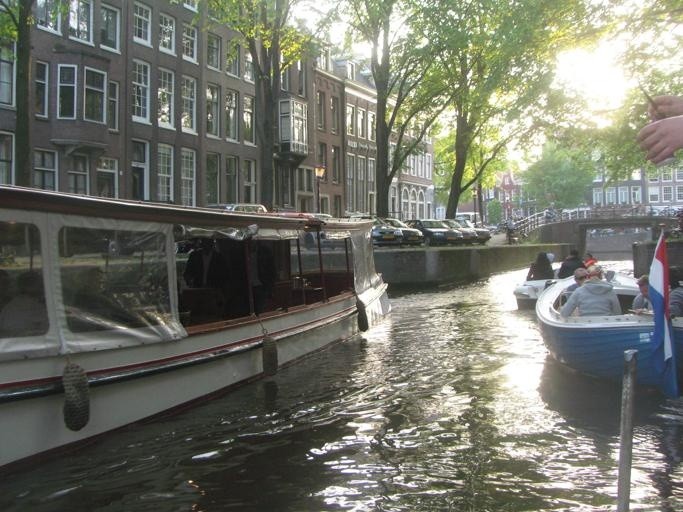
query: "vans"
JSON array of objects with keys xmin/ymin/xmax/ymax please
[
  {"xmin": 207, "ymin": 203, "xmax": 268, "ymax": 213},
  {"xmin": 456, "ymin": 211, "xmax": 480, "ymax": 227}
]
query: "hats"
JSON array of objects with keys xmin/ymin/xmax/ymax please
[{"xmin": 586, "ymin": 266, "xmax": 601, "ymax": 274}]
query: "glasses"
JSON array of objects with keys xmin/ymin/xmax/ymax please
[{"xmin": 575, "ymin": 276, "xmax": 586, "ymax": 281}]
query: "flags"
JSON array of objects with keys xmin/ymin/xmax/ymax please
[{"xmin": 643, "ymin": 235, "xmax": 679, "ymax": 400}]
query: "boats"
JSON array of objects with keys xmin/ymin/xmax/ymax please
[
  {"xmin": 0, "ymin": 180, "xmax": 394, "ymax": 471},
  {"xmin": 534, "ymin": 263, "xmax": 683, "ymax": 397},
  {"xmin": 513, "ymin": 261, "xmax": 610, "ymax": 309}
]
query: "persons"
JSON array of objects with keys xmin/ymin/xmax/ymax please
[
  {"xmin": 635, "ymin": 94, "xmax": 682, "ymax": 165},
  {"xmin": 524, "ymin": 249, "xmax": 681, "ymax": 320}
]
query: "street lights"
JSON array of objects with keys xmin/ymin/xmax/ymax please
[
  {"xmin": 470, "ymin": 186, "xmax": 479, "ymax": 227},
  {"xmin": 311, "ymin": 165, "xmax": 327, "ymax": 212}
]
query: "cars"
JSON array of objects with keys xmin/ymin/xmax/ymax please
[
  {"xmin": 463, "ymin": 220, "xmax": 492, "ymax": 244},
  {"xmin": 339, "ymin": 214, "xmax": 403, "ymax": 247},
  {"xmin": 382, "ymin": 218, "xmax": 425, "ymax": 245},
  {"xmin": 403, "ymin": 219, "xmax": 464, "ymax": 246},
  {"xmin": 441, "ymin": 220, "xmax": 478, "ymax": 244}
]
